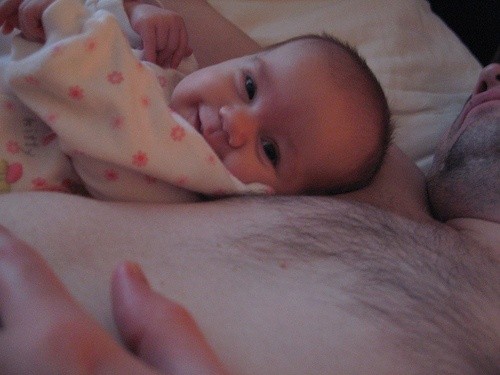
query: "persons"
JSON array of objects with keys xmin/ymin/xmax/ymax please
[
  {"xmin": 1, "ymin": 0, "xmax": 393, "ymax": 203},
  {"xmin": 0, "ymin": 0, "xmax": 499, "ymax": 375}
]
